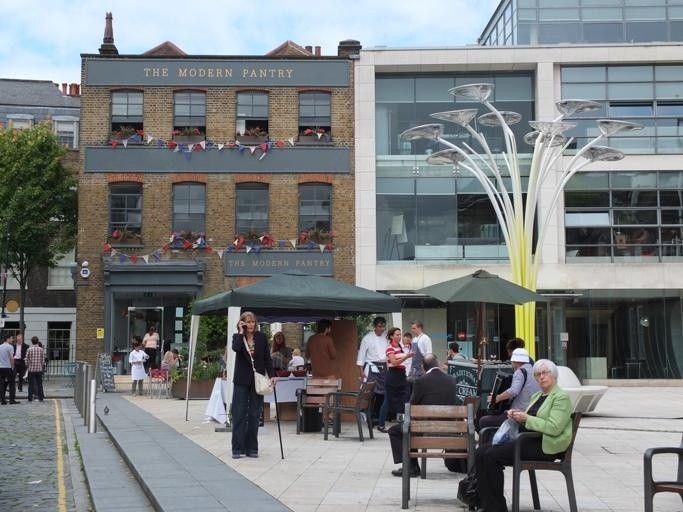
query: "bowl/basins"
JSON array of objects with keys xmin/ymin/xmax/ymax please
[
  {"xmin": 293, "ymin": 371, "xmax": 307, "ymax": 377},
  {"xmin": 276, "ymin": 371, "xmax": 291, "ymax": 377}
]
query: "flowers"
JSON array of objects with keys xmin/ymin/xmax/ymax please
[
  {"xmin": 297, "ymin": 127, "xmax": 331, "ymax": 137},
  {"xmin": 234, "ymin": 225, "xmax": 276, "ymax": 246},
  {"xmin": 169, "ymin": 126, "xmax": 202, "ymax": 137},
  {"xmin": 108, "ymin": 123, "xmax": 143, "ymax": 138},
  {"xmin": 109, "ymin": 229, "xmax": 143, "ymax": 244},
  {"xmin": 298, "ymin": 226, "xmax": 337, "ymax": 253},
  {"xmin": 244, "ymin": 125, "xmax": 268, "ymax": 138},
  {"xmin": 168, "ymin": 229, "xmax": 207, "ymax": 245}
]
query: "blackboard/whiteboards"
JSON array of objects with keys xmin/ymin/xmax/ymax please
[{"xmin": 99, "ymin": 353, "xmax": 115, "ymax": 390}]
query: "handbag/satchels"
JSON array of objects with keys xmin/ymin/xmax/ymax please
[
  {"xmin": 457, "ymin": 464, "xmax": 480, "ymax": 508},
  {"xmin": 254, "ymin": 371, "xmax": 274, "ymax": 396}
]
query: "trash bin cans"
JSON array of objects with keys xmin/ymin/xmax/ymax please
[{"xmin": 295, "ymin": 388, "xmax": 323, "ymax": 434}]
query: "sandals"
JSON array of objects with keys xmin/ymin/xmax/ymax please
[{"xmin": 322, "ymin": 417, "xmax": 333, "ymax": 428}]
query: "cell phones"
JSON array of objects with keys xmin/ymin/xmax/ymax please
[{"xmin": 241, "ymin": 325, "xmax": 247, "ymax": 331}]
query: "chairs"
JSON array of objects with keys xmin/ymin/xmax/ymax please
[
  {"xmin": 147, "ymin": 367, "xmax": 171, "ymax": 400},
  {"xmin": 322, "ymin": 379, "xmax": 377, "ymax": 442},
  {"xmin": 478, "ymin": 410, "xmax": 584, "ymax": 512},
  {"xmin": 420, "ymin": 394, "xmax": 481, "ymax": 479},
  {"xmin": 399, "ymin": 401, "xmax": 479, "ymax": 512},
  {"xmin": 639, "ymin": 430, "xmax": 683, "ymax": 512},
  {"xmin": 293, "ymin": 376, "xmax": 344, "ymax": 436}
]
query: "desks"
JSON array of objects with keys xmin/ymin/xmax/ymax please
[
  {"xmin": 205, "ymin": 374, "xmax": 313, "ymax": 425},
  {"xmin": 574, "ymin": 356, "xmax": 608, "ymax": 380}
]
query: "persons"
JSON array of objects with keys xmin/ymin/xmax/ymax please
[
  {"xmin": 129, "ymin": 342, "xmax": 149, "ymax": 397},
  {"xmin": 142, "ymin": 326, "xmax": 160, "ymax": 371},
  {"xmin": 270, "ymin": 333, "xmax": 306, "ymax": 377},
  {"xmin": 161, "ymin": 348, "xmax": 179, "ymax": 372},
  {"xmin": 305, "ymin": 318, "xmax": 336, "ymax": 428},
  {"xmin": 479, "ymin": 348, "xmax": 543, "ymax": 429},
  {"xmin": 474, "ymin": 339, "xmax": 535, "ymax": 434},
  {"xmin": 231, "ymin": 312, "xmax": 277, "ymax": 458},
  {"xmin": 388, "ymin": 355, "xmax": 456, "ymax": 478},
  {"xmin": 475, "ymin": 360, "xmax": 574, "ymax": 511},
  {"xmin": 0, "ymin": 333, "xmax": 48, "ymax": 405},
  {"xmin": 441, "ymin": 342, "xmax": 467, "ymax": 369},
  {"xmin": 355, "ymin": 317, "xmax": 433, "ymax": 434}
]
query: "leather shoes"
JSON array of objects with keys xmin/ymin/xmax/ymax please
[
  {"xmin": 392, "ymin": 466, "xmax": 420, "ymax": 478},
  {"xmin": 246, "ymin": 453, "xmax": 259, "ymax": 458},
  {"xmin": 377, "ymin": 425, "xmax": 390, "ymax": 433},
  {"xmin": 0, "ymin": 387, "xmax": 43, "ymax": 405},
  {"xmin": 399, "ymin": 465, "xmax": 422, "ymax": 476},
  {"xmin": 232, "ymin": 454, "xmax": 241, "ymax": 459}
]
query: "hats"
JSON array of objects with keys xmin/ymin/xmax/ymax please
[
  {"xmin": 511, "ymin": 348, "xmax": 529, "ymax": 364},
  {"xmin": 291, "ymin": 349, "xmax": 301, "ymax": 356}
]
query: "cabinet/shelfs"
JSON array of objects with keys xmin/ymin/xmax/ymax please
[{"xmin": 443, "ymin": 359, "xmax": 515, "ymax": 410}]
